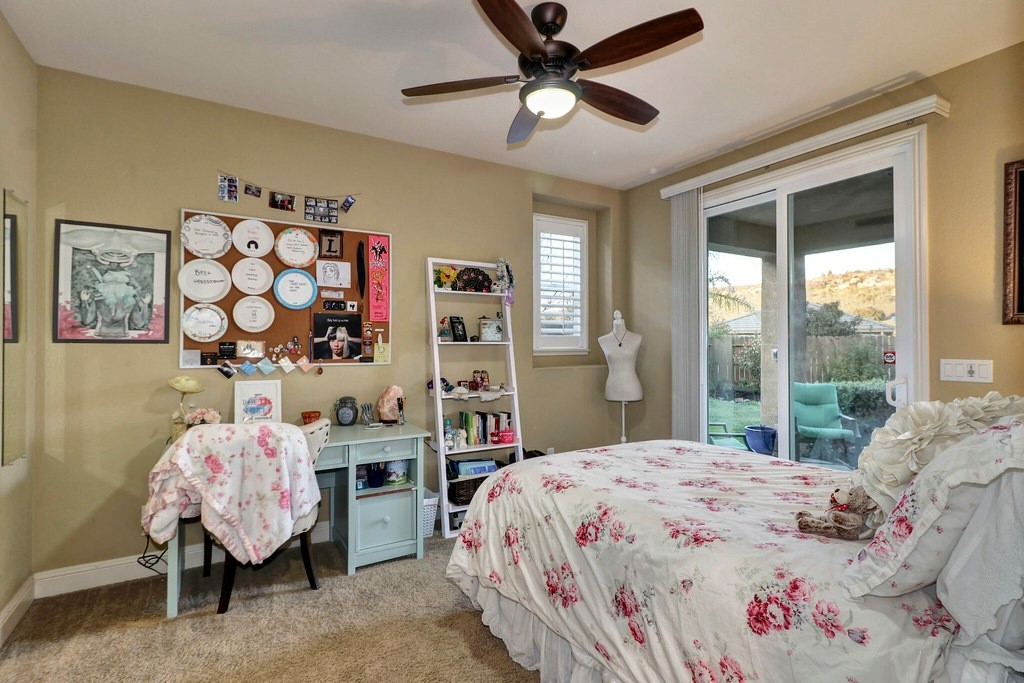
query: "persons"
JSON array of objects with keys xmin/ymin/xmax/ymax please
[
  {"xmin": 313, "ymin": 327, "xmax": 361, "ymax": 360},
  {"xmin": 438, "ymin": 317, "xmax": 452, "ymax": 341},
  {"xmin": 597, "ymin": 309, "xmax": 645, "ymax": 402}
]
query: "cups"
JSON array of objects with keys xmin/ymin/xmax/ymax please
[
  {"xmin": 301, "ymin": 411, "xmax": 321, "ymax": 425},
  {"xmin": 366, "ymin": 463, "xmax": 386, "ymax": 488}
]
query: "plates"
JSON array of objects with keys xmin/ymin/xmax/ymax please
[
  {"xmin": 273, "ymin": 269, "xmax": 318, "ymax": 310},
  {"xmin": 177, "ymin": 259, "xmax": 231, "ymax": 304},
  {"xmin": 273, "ymin": 227, "xmax": 319, "ymax": 269},
  {"xmin": 232, "ymin": 258, "xmax": 274, "ymax": 295},
  {"xmin": 182, "ymin": 304, "xmax": 228, "ymax": 343},
  {"xmin": 231, "ymin": 220, "xmax": 275, "ymax": 257},
  {"xmin": 180, "ymin": 215, "xmax": 232, "ymax": 259},
  {"xmin": 233, "ymin": 296, "xmax": 275, "ymax": 333}
]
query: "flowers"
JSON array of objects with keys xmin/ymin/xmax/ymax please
[{"xmin": 184, "ymin": 407, "xmax": 222, "ymax": 425}]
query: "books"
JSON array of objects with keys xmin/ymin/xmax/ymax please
[{"xmin": 459, "ymin": 410, "xmax": 511, "ymax": 446}]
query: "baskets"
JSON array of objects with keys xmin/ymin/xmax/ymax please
[
  {"xmin": 448, "ymin": 458, "xmax": 508, "ymax": 507},
  {"xmin": 423, "ymin": 486, "xmax": 440, "ymax": 538}
]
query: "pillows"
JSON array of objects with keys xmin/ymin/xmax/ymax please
[{"xmin": 839, "ymin": 414, "xmax": 1024, "ymax": 673}]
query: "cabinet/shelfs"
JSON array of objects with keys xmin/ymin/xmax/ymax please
[{"xmin": 425, "ymin": 257, "xmax": 525, "ymax": 539}]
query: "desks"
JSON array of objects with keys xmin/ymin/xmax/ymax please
[{"xmin": 166, "ymin": 422, "xmax": 433, "ymax": 622}]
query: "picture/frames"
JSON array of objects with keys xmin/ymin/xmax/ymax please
[
  {"xmin": 1002, "ymin": 159, "xmax": 1024, "ymax": 325},
  {"xmin": 234, "ymin": 380, "xmax": 283, "ymax": 423},
  {"xmin": 450, "ymin": 316, "xmax": 468, "ymax": 342},
  {"xmin": 3, "ymin": 215, "xmax": 19, "ymax": 343},
  {"xmin": 52, "ymin": 218, "xmax": 172, "ymax": 344},
  {"xmin": 319, "ymin": 229, "xmax": 344, "ymax": 258}
]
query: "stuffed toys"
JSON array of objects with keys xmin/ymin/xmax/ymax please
[{"xmin": 796, "ymin": 485, "xmax": 878, "ymax": 542}]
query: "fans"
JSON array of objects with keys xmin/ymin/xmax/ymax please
[{"xmin": 401, "ymin": 0, "xmax": 704, "ymax": 144}]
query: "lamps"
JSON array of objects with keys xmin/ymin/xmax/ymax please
[
  {"xmin": 519, "ymin": 67, "xmax": 583, "ymax": 120},
  {"xmin": 167, "ymin": 375, "xmax": 206, "ymax": 442}
]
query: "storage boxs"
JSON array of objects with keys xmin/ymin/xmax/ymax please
[{"xmin": 479, "ymin": 320, "xmax": 504, "ymax": 341}]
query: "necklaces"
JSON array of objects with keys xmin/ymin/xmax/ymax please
[{"xmin": 612, "ymin": 329, "xmax": 628, "ymax": 347}]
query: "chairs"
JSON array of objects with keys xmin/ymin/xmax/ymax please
[
  {"xmin": 785, "ymin": 380, "xmax": 862, "ymax": 471},
  {"xmin": 708, "ymin": 422, "xmax": 757, "ymax": 453},
  {"xmin": 201, "ymin": 417, "xmax": 332, "ymax": 615}
]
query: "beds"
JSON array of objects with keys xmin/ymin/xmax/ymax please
[{"xmin": 441, "ymin": 438, "xmax": 1024, "ymax": 683}]
